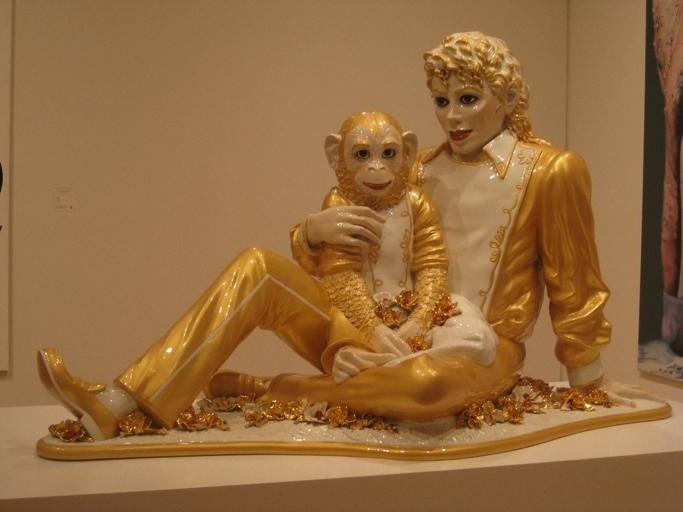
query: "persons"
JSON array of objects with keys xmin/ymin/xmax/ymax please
[{"xmin": 37, "ymin": 29, "xmax": 665, "ymax": 444}]
